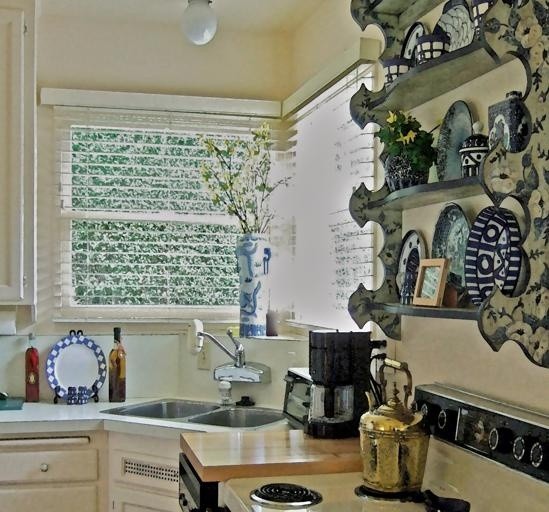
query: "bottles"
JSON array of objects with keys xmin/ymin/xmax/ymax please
[
  {"xmin": 218, "ymin": 382, "xmax": 232, "ymax": 405},
  {"xmin": 67, "ymin": 386, "xmax": 87, "ymax": 405},
  {"xmin": 25, "ymin": 332, "xmax": 39, "ymax": 402},
  {"xmin": 266, "ymin": 310, "xmax": 278, "ymax": 336},
  {"xmin": 110, "ymin": 327, "xmax": 126, "ymax": 400}
]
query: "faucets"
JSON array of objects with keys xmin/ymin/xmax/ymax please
[{"xmin": 196, "ymin": 330, "xmax": 245, "ymax": 366}]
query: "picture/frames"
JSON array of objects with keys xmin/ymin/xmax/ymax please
[{"xmin": 412, "ymin": 258, "xmax": 450, "ymax": 307}]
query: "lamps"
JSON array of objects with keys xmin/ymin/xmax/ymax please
[{"xmin": 181, "ymin": 0, "xmax": 219, "ymax": 45}]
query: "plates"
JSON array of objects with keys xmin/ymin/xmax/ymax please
[
  {"xmin": 432, "ymin": 5, "xmax": 474, "ymax": 50},
  {"xmin": 432, "ymin": 203, "xmax": 473, "ymax": 307},
  {"xmin": 467, "ymin": 206, "xmax": 522, "ymax": 306},
  {"xmin": 435, "ymin": 100, "xmax": 473, "ymax": 180},
  {"xmin": 45, "ymin": 336, "xmax": 107, "ymax": 401},
  {"xmin": 395, "ymin": 231, "xmax": 426, "ymax": 301},
  {"xmin": 399, "ymin": 23, "xmax": 424, "ymax": 58}
]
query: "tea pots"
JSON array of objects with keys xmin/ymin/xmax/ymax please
[{"xmin": 359, "ymin": 359, "xmax": 431, "ymax": 489}]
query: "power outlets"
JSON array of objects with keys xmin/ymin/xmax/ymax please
[
  {"xmin": 196, "ymin": 343, "xmax": 212, "ymax": 369},
  {"xmin": 376, "ymin": 341, "xmax": 396, "ymax": 374}
]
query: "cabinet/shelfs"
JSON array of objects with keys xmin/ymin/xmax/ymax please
[
  {"xmin": 0, "ymin": 0, "xmax": 38, "ymax": 305},
  {"xmin": 342, "ymin": 0, "xmax": 549, "ymax": 369},
  {"xmin": 0, "ymin": 434, "xmax": 106, "ymax": 512}
]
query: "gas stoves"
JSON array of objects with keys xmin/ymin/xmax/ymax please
[{"xmin": 225, "ymin": 471, "xmax": 486, "ymax": 512}]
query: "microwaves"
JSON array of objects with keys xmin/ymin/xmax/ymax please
[{"xmin": 281, "ymin": 367, "xmax": 313, "ymax": 429}]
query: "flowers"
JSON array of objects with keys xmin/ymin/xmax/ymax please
[
  {"xmin": 372, "ymin": 108, "xmax": 444, "ymax": 171},
  {"xmin": 195, "ymin": 122, "xmax": 298, "ymax": 236}
]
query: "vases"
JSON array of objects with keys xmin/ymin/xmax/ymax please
[
  {"xmin": 384, "ymin": 153, "xmax": 429, "ymax": 192},
  {"xmin": 236, "ymin": 237, "xmax": 271, "ymax": 337}
]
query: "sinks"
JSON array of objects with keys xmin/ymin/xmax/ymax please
[
  {"xmin": 99, "ymin": 399, "xmax": 217, "ymax": 423},
  {"xmin": 189, "ymin": 408, "xmax": 287, "ymax": 429}
]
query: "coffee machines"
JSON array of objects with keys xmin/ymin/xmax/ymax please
[{"xmin": 304, "ymin": 328, "xmax": 372, "ymax": 437}]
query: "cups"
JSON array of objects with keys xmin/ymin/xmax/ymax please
[
  {"xmin": 381, "ymin": 59, "xmax": 414, "ymax": 85},
  {"xmin": 400, "ymin": 271, "xmax": 418, "ymax": 304},
  {"xmin": 414, "ymin": 35, "xmax": 451, "ymax": 61}
]
